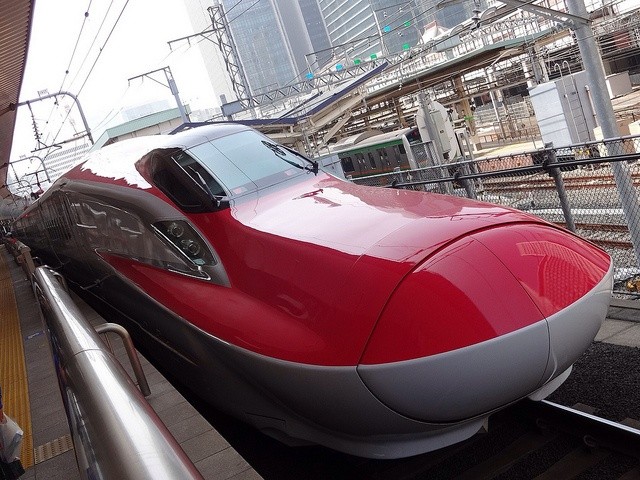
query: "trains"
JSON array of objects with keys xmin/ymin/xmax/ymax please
[
  {"xmin": 315, "ymin": 124, "xmax": 430, "ymax": 187},
  {"xmin": 9, "ymin": 122, "xmax": 617, "ymax": 460}
]
